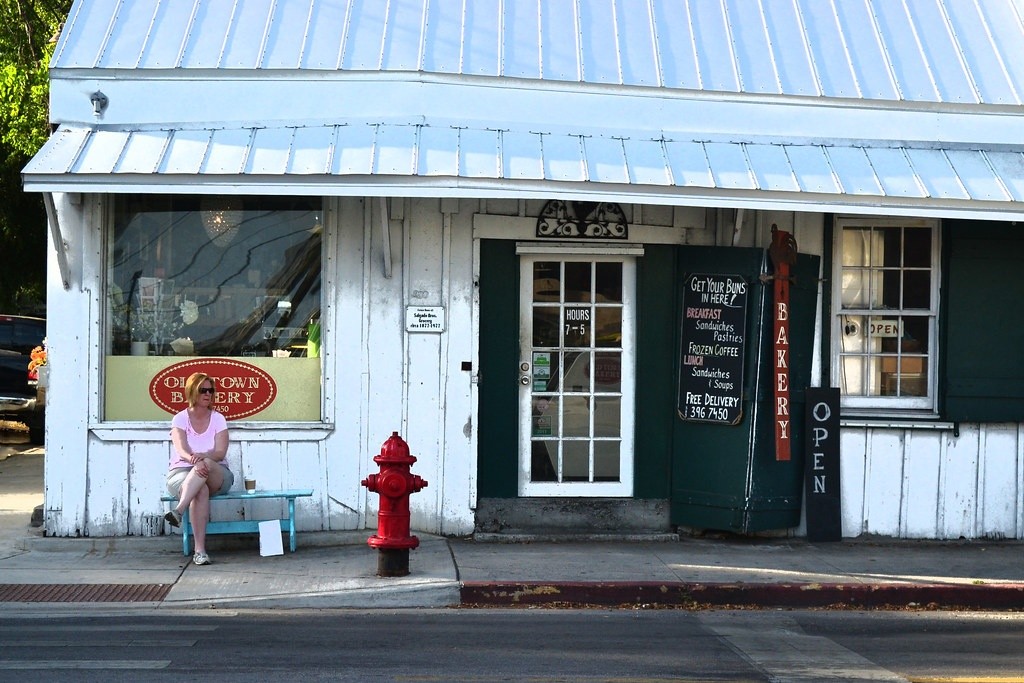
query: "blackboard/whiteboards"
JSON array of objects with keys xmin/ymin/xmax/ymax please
[
  {"xmin": 676, "ymin": 272, "xmax": 749, "ymax": 427},
  {"xmin": 803, "ymin": 385, "xmax": 843, "ymax": 542}
]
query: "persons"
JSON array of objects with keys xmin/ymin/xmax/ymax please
[{"xmin": 162, "ymin": 372, "xmax": 235, "ymax": 567}]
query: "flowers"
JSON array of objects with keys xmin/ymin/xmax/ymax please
[{"xmin": 27, "ymin": 337, "xmax": 47, "ymax": 380}]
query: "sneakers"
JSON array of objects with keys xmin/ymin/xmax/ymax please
[
  {"xmin": 164, "ymin": 509, "xmax": 182, "ymax": 528},
  {"xmin": 192, "ymin": 552, "xmax": 211, "ymax": 566}
]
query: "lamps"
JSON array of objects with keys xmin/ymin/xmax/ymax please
[{"xmin": 200, "ymin": 211, "xmax": 243, "ymax": 247}]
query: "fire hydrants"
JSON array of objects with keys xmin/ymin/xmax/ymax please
[{"xmin": 361, "ymin": 432, "xmax": 429, "ymax": 578}]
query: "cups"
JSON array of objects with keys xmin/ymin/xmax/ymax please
[{"xmin": 244, "ymin": 478, "xmax": 256, "ymax": 494}]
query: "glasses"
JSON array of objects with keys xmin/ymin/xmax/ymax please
[{"xmin": 197, "ymin": 387, "xmax": 215, "ymax": 394}]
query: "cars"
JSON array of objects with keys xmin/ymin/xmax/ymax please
[{"xmin": 0, "ymin": 315, "xmax": 46, "ymax": 426}]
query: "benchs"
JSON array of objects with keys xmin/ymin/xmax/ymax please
[{"xmin": 160, "ymin": 487, "xmax": 314, "ymax": 556}]
